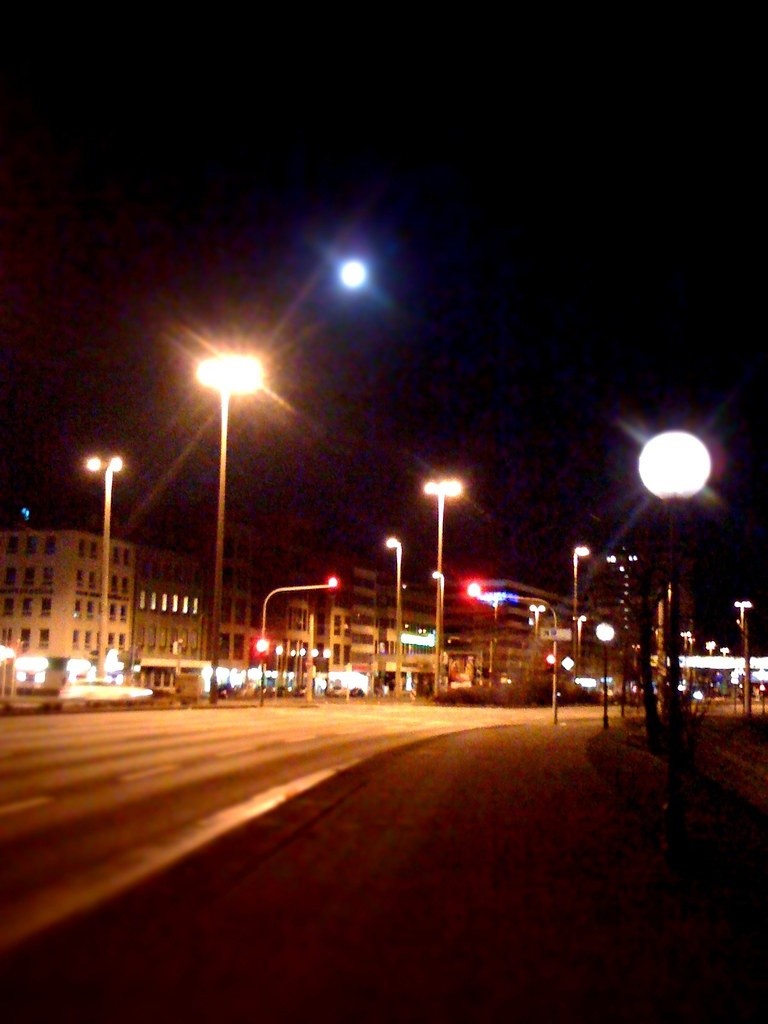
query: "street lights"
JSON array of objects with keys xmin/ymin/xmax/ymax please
[
  {"xmin": 87, "ymin": 455, "xmax": 123, "ymax": 677},
  {"xmin": 386, "ymin": 538, "xmax": 402, "ymax": 697},
  {"xmin": 574, "ymin": 547, "xmax": 591, "ymax": 661},
  {"xmin": 424, "ymin": 480, "xmax": 461, "ymax": 694},
  {"xmin": 734, "ymin": 601, "xmax": 754, "ymax": 713},
  {"xmin": 431, "ymin": 572, "xmax": 444, "ymax": 685}
]
